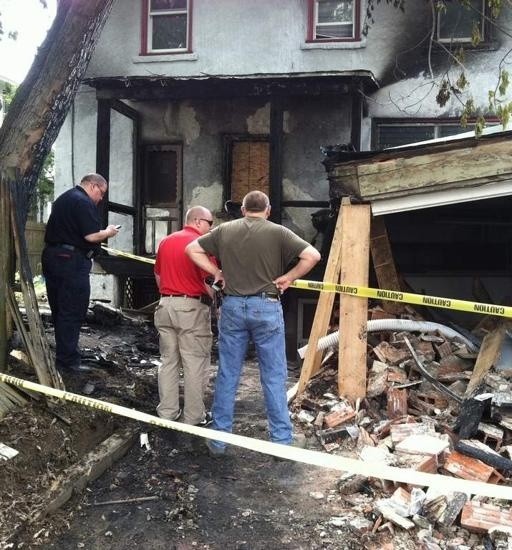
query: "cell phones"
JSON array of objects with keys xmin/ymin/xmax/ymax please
[{"xmin": 115, "ymin": 224, "xmax": 121, "ymax": 229}]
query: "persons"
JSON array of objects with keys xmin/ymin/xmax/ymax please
[
  {"xmin": 40, "ymin": 172, "xmax": 121, "ymax": 373},
  {"xmin": 182, "ymin": 190, "xmax": 324, "ymax": 463},
  {"xmin": 152, "ymin": 204, "xmax": 218, "ymax": 428}
]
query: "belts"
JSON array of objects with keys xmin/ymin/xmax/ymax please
[
  {"xmin": 223, "ymin": 290, "xmax": 281, "ymax": 300},
  {"xmin": 159, "ymin": 292, "xmax": 203, "ymax": 300}
]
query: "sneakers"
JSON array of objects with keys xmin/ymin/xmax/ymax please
[
  {"xmin": 186, "ymin": 410, "xmax": 214, "ymax": 428},
  {"xmin": 270, "ymin": 432, "xmax": 307, "ymax": 462}
]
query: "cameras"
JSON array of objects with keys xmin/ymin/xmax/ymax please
[{"xmin": 212, "ymin": 284, "xmax": 222, "ymax": 291}]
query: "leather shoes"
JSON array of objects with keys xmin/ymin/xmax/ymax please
[
  {"xmin": 54, "ymin": 363, "xmax": 93, "ymax": 374},
  {"xmin": 49, "ymin": 241, "xmax": 88, "ymax": 259}
]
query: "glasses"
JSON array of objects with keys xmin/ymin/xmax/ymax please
[{"xmin": 198, "ymin": 217, "xmax": 215, "ymax": 228}]
